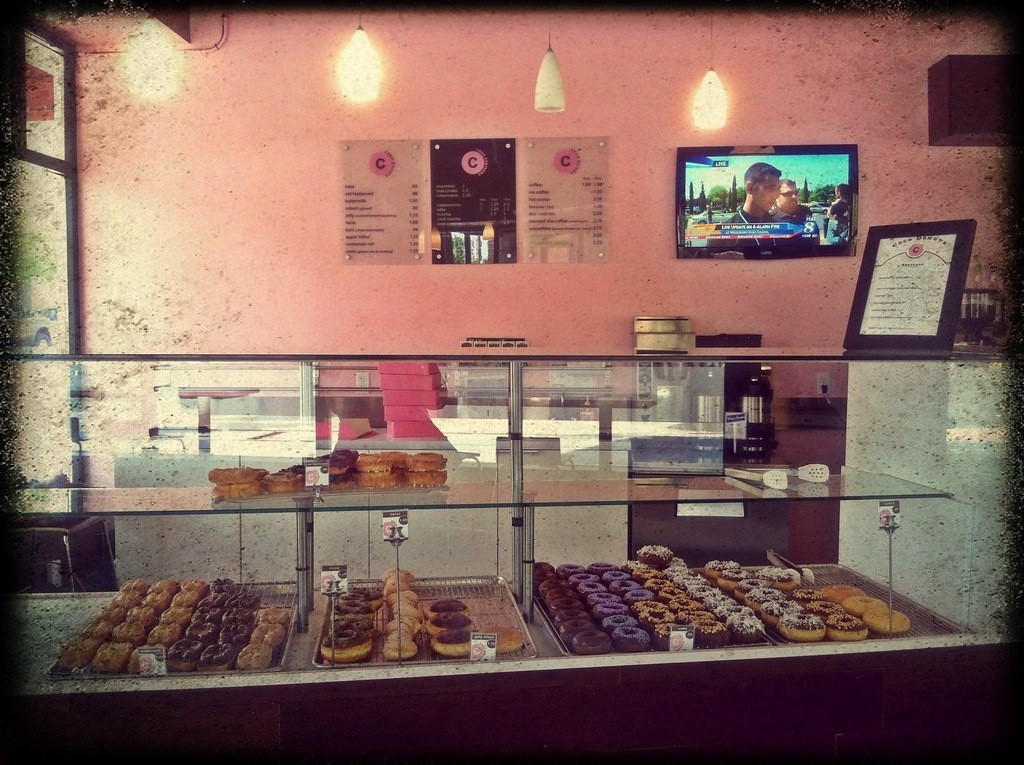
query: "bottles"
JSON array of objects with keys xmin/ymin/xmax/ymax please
[
  {"xmin": 954, "ymin": 261, "xmax": 1006, "ymax": 345},
  {"xmin": 482, "ymin": 223, "xmax": 495, "ymax": 262}
]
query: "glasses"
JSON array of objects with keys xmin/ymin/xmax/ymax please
[{"xmin": 778, "ymin": 190, "xmax": 799, "ymax": 197}]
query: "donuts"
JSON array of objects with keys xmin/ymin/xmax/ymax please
[
  {"xmin": 320, "ymin": 586, "xmax": 384, "ymax": 663},
  {"xmin": 423, "ymin": 597, "xmax": 525, "ymax": 657},
  {"xmin": 208, "ymin": 448, "xmax": 448, "ymax": 500},
  {"xmin": 533, "ymin": 542, "xmax": 911, "ymax": 656}
]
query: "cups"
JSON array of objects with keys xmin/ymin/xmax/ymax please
[
  {"xmin": 451, "ymin": 232, "xmax": 466, "ymax": 264},
  {"xmin": 469, "ymin": 234, "xmax": 483, "ymax": 264}
]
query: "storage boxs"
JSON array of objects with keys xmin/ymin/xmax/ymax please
[
  {"xmin": 383, "ymin": 385, "xmax": 446, "ymax": 405},
  {"xmin": 316, "ymin": 417, "xmax": 371, "ymax": 439},
  {"xmin": 378, "ymin": 361, "xmax": 443, "ymax": 375},
  {"xmin": 381, "ymin": 372, "xmax": 442, "ymax": 391},
  {"xmin": 387, "ymin": 421, "xmax": 445, "ymax": 436},
  {"xmin": 384, "ymin": 405, "xmax": 443, "ymax": 422}
]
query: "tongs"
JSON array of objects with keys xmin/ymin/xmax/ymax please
[
  {"xmin": 764, "ymin": 547, "xmax": 816, "ymax": 584},
  {"xmin": 722, "ymin": 463, "xmax": 831, "ymax": 489}
]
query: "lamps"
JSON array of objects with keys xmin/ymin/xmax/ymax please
[
  {"xmin": 337, "ymin": 13, "xmax": 378, "ymax": 101},
  {"xmin": 692, "ymin": 11, "xmax": 728, "ymax": 128},
  {"xmin": 535, "ymin": 13, "xmax": 567, "ymax": 113}
]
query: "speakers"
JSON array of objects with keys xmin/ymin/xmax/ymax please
[{"xmin": 925, "ymin": 52, "xmax": 1024, "ymax": 146}]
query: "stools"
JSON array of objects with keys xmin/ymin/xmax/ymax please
[{"xmin": 4, "ymin": 516, "xmax": 114, "ymax": 593}]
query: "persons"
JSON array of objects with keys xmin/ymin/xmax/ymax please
[{"xmin": 705, "ymin": 162, "xmax": 849, "ymax": 246}]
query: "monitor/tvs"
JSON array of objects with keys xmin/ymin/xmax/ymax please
[{"xmin": 674, "ymin": 143, "xmax": 858, "ymax": 258}]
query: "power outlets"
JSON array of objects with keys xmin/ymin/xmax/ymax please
[
  {"xmin": 460, "ymin": 340, "xmax": 528, "ymax": 348},
  {"xmin": 816, "ymin": 371, "xmax": 831, "ymax": 395},
  {"xmin": 356, "ymin": 372, "xmax": 369, "ymax": 393}
]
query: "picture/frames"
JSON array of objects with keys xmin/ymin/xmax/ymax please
[{"xmin": 843, "ymin": 219, "xmax": 979, "ymax": 352}]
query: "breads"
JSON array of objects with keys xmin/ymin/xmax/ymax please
[
  {"xmin": 58, "ymin": 573, "xmax": 291, "ymax": 673},
  {"xmin": 381, "ymin": 567, "xmax": 422, "ymax": 660}
]
